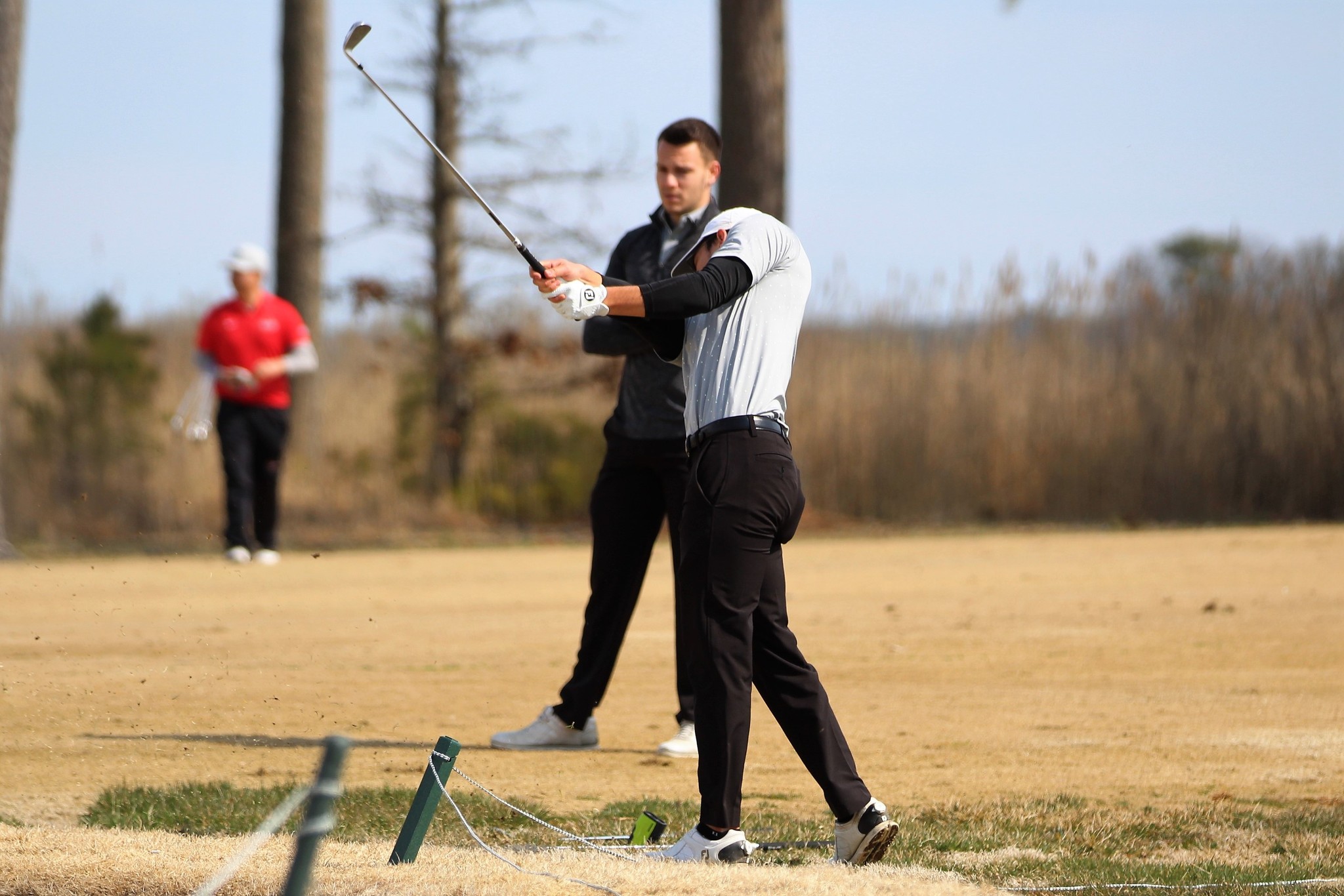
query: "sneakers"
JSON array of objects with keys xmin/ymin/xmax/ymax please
[
  {"xmin": 643, "ymin": 823, "xmax": 749, "ymax": 867},
  {"xmin": 658, "ymin": 720, "xmax": 699, "ymax": 759},
  {"xmin": 491, "ymin": 706, "xmax": 599, "ymax": 749},
  {"xmin": 827, "ymin": 797, "xmax": 899, "ymax": 866}
]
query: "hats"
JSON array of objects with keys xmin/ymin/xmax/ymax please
[
  {"xmin": 670, "ymin": 207, "xmax": 762, "ymax": 278},
  {"xmin": 226, "ymin": 244, "xmax": 266, "ymax": 270}
]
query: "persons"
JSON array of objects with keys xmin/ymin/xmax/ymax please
[
  {"xmin": 192, "ymin": 243, "xmax": 320, "ymax": 565},
  {"xmin": 538, "ymin": 204, "xmax": 897, "ymax": 866},
  {"xmin": 487, "ymin": 117, "xmax": 725, "ymax": 761}
]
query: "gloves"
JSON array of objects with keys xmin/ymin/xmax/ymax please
[{"xmin": 541, "ymin": 280, "xmax": 609, "ymax": 319}]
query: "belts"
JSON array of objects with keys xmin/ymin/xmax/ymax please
[{"xmin": 685, "ymin": 415, "xmax": 789, "ymax": 454}]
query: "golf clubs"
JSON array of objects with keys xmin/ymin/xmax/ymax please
[{"xmin": 341, "ymin": 21, "xmax": 582, "ymax": 322}]
